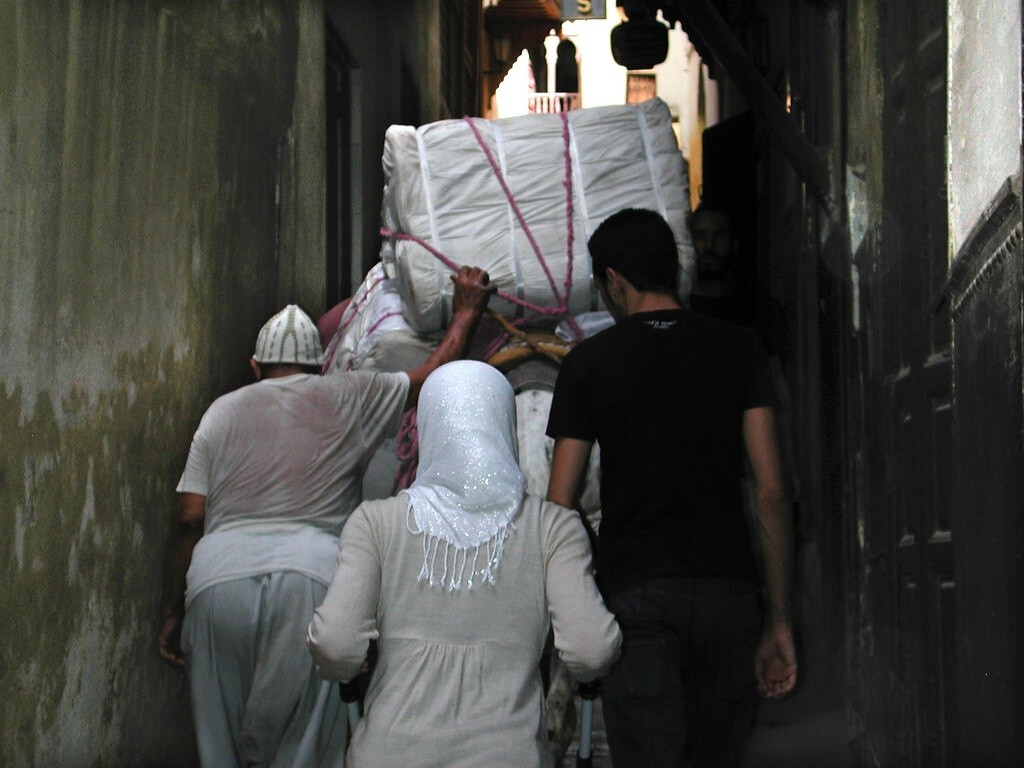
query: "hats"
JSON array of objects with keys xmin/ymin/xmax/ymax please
[{"xmin": 253, "ymin": 304, "xmax": 325, "ymax": 366}]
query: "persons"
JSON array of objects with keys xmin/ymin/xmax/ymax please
[
  {"xmin": 157, "ymin": 263, "xmax": 499, "ymax": 768},
  {"xmin": 544, "ymin": 197, "xmax": 799, "ymax": 768},
  {"xmin": 304, "ymin": 356, "xmax": 625, "ymax": 768}
]
